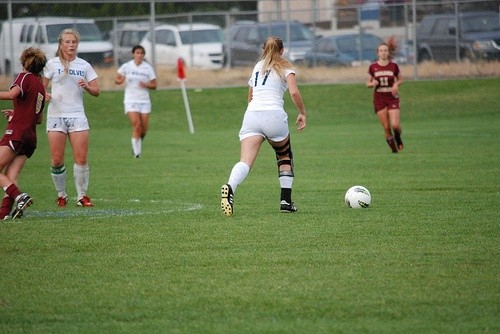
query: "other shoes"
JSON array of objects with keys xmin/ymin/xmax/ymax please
[
  {"xmin": 385, "ymin": 137, "xmax": 399, "ymax": 153},
  {"xmin": 394, "ymin": 133, "xmax": 404, "ymax": 150},
  {"xmin": 221, "ymin": 183, "xmax": 235, "ymax": 217}
]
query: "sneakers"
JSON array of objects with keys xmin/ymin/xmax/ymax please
[
  {"xmin": 280, "ymin": 200, "xmax": 299, "ymax": 212},
  {"xmin": 11, "ymin": 195, "xmax": 32, "ymax": 221},
  {"xmin": 75, "ymin": 196, "xmax": 95, "ymax": 207},
  {"xmin": 56, "ymin": 194, "xmax": 69, "ymax": 208}
]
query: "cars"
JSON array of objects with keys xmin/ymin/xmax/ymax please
[
  {"xmin": 137, "ymin": 23, "xmax": 226, "ymax": 71},
  {"xmin": 102, "ymin": 21, "xmax": 166, "ymax": 64},
  {"xmin": 304, "ymin": 34, "xmax": 407, "ymax": 69}
]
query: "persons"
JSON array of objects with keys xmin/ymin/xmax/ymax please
[
  {"xmin": 366, "ymin": 35, "xmax": 403, "ymax": 153},
  {"xmin": 115, "ymin": 45, "xmax": 157, "ymax": 158},
  {"xmin": 0, "ymin": 48, "xmax": 47, "ymax": 222},
  {"xmin": 220, "ymin": 37, "xmax": 307, "ymax": 217},
  {"xmin": 40, "ymin": 29, "xmax": 100, "ymax": 207}
]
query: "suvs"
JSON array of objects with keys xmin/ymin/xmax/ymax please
[
  {"xmin": 226, "ymin": 21, "xmax": 323, "ymax": 67},
  {"xmin": 415, "ymin": 12, "xmax": 500, "ymax": 64}
]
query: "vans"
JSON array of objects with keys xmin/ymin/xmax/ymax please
[{"xmin": 0, "ymin": 16, "xmax": 115, "ymax": 83}]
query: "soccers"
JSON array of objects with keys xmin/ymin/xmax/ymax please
[{"xmin": 345, "ymin": 185, "xmax": 371, "ymax": 209}]
